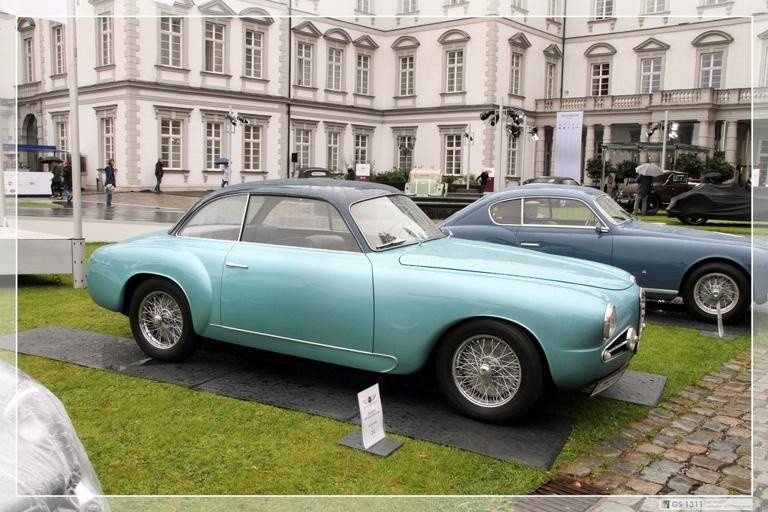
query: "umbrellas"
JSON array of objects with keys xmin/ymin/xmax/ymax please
[
  {"xmin": 635, "ymin": 163, "xmax": 665, "ymax": 178},
  {"xmin": 213, "ymin": 158, "xmax": 232, "ymax": 165},
  {"xmin": 104, "ymin": 184, "xmax": 115, "ymax": 195},
  {"xmin": 39, "ymin": 156, "xmax": 63, "ymax": 168}
]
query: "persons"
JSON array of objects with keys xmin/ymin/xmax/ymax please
[
  {"xmin": 221, "ymin": 162, "xmax": 231, "ymax": 188},
  {"xmin": 51, "ymin": 162, "xmax": 62, "ymax": 199},
  {"xmin": 154, "ymin": 158, "xmax": 164, "ymax": 194},
  {"xmin": 62, "ymin": 159, "xmax": 73, "ymax": 202},
  {"xmin": 105, "ymin": 157, "xmax": 117, "ymax": 210},
  {"xmin": 476, "ymin": 169, "xmax": 488, "ymax": 198},
  {"xmin": 630, "ymin": 174, "xmax": 652, "ymax": 216},
  {"xmin": 604, "ymin": 172, "xmax": 616, "ymax": 198},
  {"xmin": 344, "ymin": 166, "xmax": 357, "ymax": 181}
]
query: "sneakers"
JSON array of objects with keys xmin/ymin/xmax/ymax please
[{"xmin": 51, "ymin": 195, "xmax": 73, "ymax": 201}]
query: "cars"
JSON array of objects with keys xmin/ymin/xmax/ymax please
[
  {"xmin": 86, "ymin": 167, "xmax": 645, "ymax": 423},
  {"xmin": 404, "ymin": 170, "xmax": 768, "ymax": 323},
  {"xmin": 1, "ymin": 362, "xmax": 105, "ymax": 512}
]
